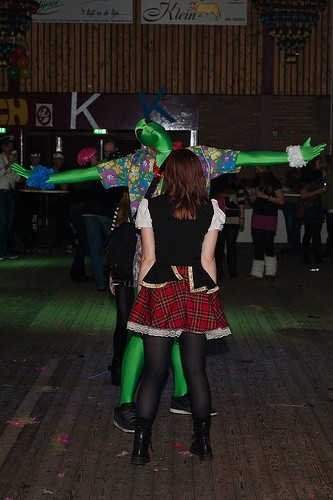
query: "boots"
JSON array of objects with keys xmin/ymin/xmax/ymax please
[
  {"xmin": 265, "ymin": 254, "xmax": 278, "ymax": 280},
  {"xmin": 131, "ymin": 416, "xmax": 154, "ymax": 464},
  {"xmin": 107, "ymin": 356, "xmax": 123, "ymax": 386},
  {"xmin": 246, "ymin": 260, "xmax": 265, "ymax": 281},
  {"xmin": 190, "ymin": 417, "xmax": 213, "ymax": 461}
]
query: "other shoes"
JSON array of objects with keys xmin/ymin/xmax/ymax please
[
  {"xmin": 98, "ymin": 286, "xmax": 111, "ymax": 292},
  {"xmin": 305, "ymin": 263, "xmax": 320, "ymax": 272},
  {"xmin": 82, "ymin": 274, "xmax": 94, "ymax": 281}
]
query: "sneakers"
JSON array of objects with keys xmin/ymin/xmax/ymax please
[
  {"xmin": 171, "ymin": 392, "xmax": 218, "ymax": 416},
  {"xmin": 113, "ymin": 402, "xmax": 151, "ymax": 432}
]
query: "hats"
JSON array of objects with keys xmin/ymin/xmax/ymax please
[
  {"xmin": 0, "ymin": 136, "xmax": 16, "ymax": 144},
  {"xmin": 77, "ymin": 148, "xmax": 97, "ymax": 165}
]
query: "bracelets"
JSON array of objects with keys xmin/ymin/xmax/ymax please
[
  {"xmin": 285, "ymin": 144, "xmax": 308, "ymax": 169},
  {"xmin": 268, "ymin": 194, "xmax": 272, "ymax": 202},
  {"xmin": 239, "ymin": 216, "xmax": 245, "ymax": 220},
  {"xmin": 26, "ymin": 166, "xmax": 55, "ymax": 191}
]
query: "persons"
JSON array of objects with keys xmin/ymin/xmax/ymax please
[
  {"xmin": 131, "ymin": 148, "xmax": 232, "ymax": 465},
  {"xmin": 0, "ymin": 133, "xmax": 333, "ymax": 386},
  {"xmin": 11, "ymin": 118, "xmax": 326, "ymax": 431}
]
